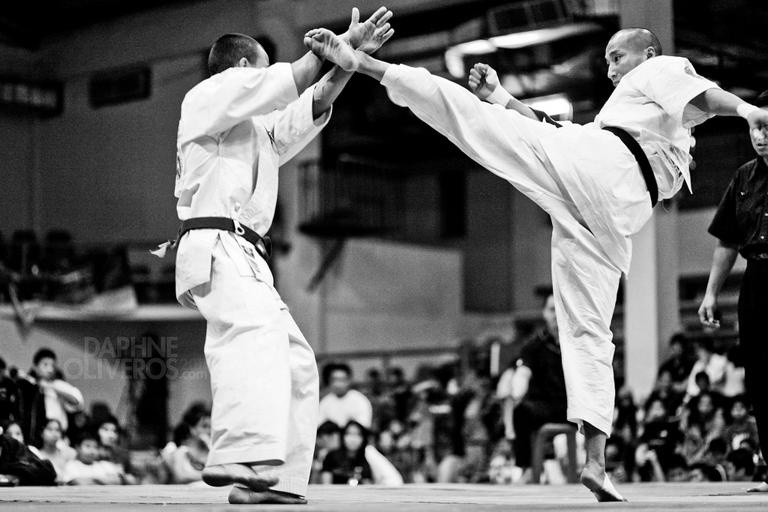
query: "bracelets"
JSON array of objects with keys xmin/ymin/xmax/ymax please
[
  {"xmin": 734, "ymin": 102, "xmax": 759, "ymax": 119},
  {"xmin": 485, "ymin": 84, "xmax": 514, "ymax": 109}
]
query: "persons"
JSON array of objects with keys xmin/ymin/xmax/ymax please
[
  {"xmin": 299, "ymin": 25, "xmax": 767, "ymax": 504},
  {"xmin": 696, "ymin": 93, "xmax": 768, "ymax": 494},
  {"xmin": 2, "ymin": 254, "xmax": 765, "ymax": 487},
  {"xmin": 149, "ymin": 4, "xmax": 398, "ymax": 507}
]
